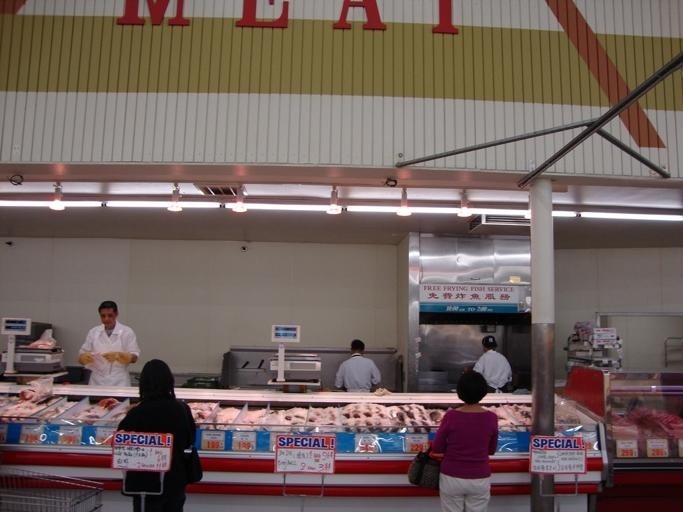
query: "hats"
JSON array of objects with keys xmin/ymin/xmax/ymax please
[{"xmin": 482, "ymin": 334, "xmax": 498, "ymax": 349}]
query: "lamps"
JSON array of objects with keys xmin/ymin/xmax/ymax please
[
  {"xmin": 524, "ymin": 195, "xmax": 532, "ymax": 219},
  {"xmin": 457, "ymin": 193, "xmax": 472, "ymax": 218},
  {"xmin": 232, "ymin": 190, "xmax": 248, "ymax": 213},
  {"xmin": 395, "ymin": 190, "xmax": 413, "ymax": 218},
  {"xmin": 0, "ymin": 200, "xmax": 102, "ymax": 210},
  {"xmin": 224, "ymin": 202, "xmax": 326, "ymax": 214},
  {"xmin": 48, "ymin": 186, "xmax": 65, "ymax": 212},
  {"xmin": 325, "ymin": 189, "xmax": 342, "ymax": 215},
  {"xmin": 168, "ymin": 189, "xmax": 184, "ymax": 213},
  {"xmin": 346, "ymin": 203, "xmax": 525, "ymax": 219},
  {"xmin": 103, "ymin": 200, "xmax": 222, "ymax": 210}
]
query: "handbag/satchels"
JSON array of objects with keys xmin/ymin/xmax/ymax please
[
  {"xmin": 407, "ymin": 444, "xmax": 440, "ymax": 493},
  {"xmin": 159, "ymin": 444, "xmax": 203, "ymax": 488}
]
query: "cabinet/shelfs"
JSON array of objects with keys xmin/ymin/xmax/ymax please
[{"xmin": 0, "ymin": 379, "xmax": 683, "ymax": 512}]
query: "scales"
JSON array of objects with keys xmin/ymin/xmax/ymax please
[
  {"xmin": 0, "ymin": 317, "xmax": 68, "ymax": 377},
  {"xmin": 269, "ymin": 324, "xmax": 322, "ymax": 385}
]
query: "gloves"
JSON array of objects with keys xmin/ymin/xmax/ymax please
[
  {"xmin": 100, "ymin": 351, "xmax": 132, "ymax": 364},
  {"xmin": 77, "ymin": 349, "xmax": 96, "ymax": 365}
]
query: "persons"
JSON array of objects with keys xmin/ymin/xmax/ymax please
[
  {"xmin": 120, "ymin": 359, "xmax": 203, "ymax": 511},
  {"xmin": 76, "ymin": 301, "xmax": 140, "ymax": 387},
  {"xmin": 334, "ymin": 339, "xmax": 381, "ymax": 392},
  {"xmin": 430, "ymin": 370, "xmax": 497, "ymax": 511},
  {"xmin": 463, "ymin": 336, "xmax": 512, "ymax": 392}
]
